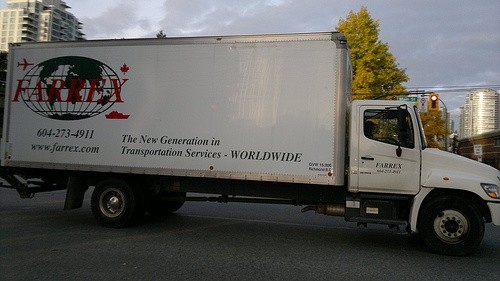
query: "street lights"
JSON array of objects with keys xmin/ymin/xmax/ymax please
[{"xmin": 421, "ymin": 94, "xmax": 447, "ymax": 150}]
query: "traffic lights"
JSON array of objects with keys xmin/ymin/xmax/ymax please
[{"xmin": 430, "ymin": 94, "xmax": 438, "ymax": 109}]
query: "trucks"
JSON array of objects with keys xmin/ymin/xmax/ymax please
[{"xmin": 0, "ymin": 31, "xmax": 500, "ymax": 257}]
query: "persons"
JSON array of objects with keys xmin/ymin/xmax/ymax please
[{"xmin": 364, "ymin": 121, "xmax": 379, "ymax": 140}]
